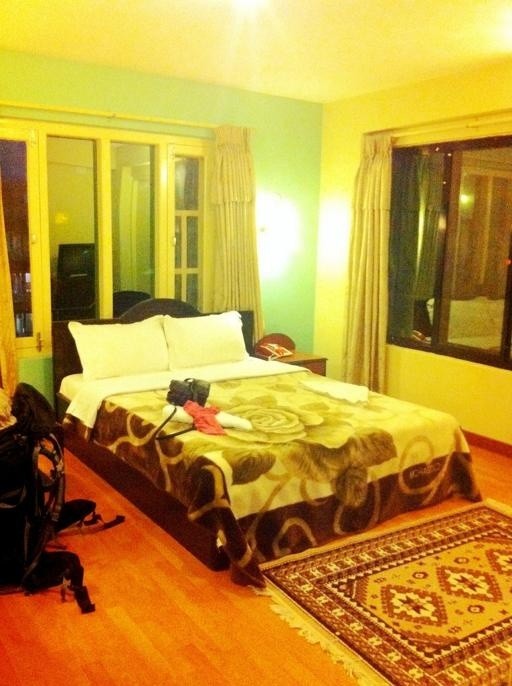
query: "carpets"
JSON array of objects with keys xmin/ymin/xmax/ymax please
[{"xmin": 256, "ymin": 496, "xmax": 512, "ymax": 686}]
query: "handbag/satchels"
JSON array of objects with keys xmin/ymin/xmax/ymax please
[{"xmin": 166, "ymin": 377, "xmax": 212, "ymax": 408}]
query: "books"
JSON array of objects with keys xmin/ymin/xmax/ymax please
[{"xmin": 261, "ymin": 342, "xmax": 293, "ymax": 357}]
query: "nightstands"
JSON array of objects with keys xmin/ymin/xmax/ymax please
[{"xmin": 253, "ymin": 334, "xmax": 328, "ymax": 377}]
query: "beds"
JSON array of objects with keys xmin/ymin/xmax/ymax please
[
  {"xmin": 53, "ymin": 298, "xmax": 482, "ymax": 587},
  {"xmin": 52, "ymin": 291, "xmax": 150, "ymax": 318},
  {"xmin": 413, "ymin": 300, "xmax": 505, "ymax": 353}
]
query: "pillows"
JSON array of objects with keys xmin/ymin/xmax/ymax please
[
  {"xmin": 488, "ymin": 299, "xmax": 505, "ymax": 334},
  {"xmin": 426, "ymin": 295, "xmax": 490, "ymax": 339},
  {"xmin": 162, "ymin": 312, "xmax": 246, "ymax": 370},
  {"xmin": 68, "ymin": 315, "xmax": 169, "ymax": 380}
]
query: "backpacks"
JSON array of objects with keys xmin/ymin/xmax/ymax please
[{"xmin": 0, "ymin": 416, "xmax": 66, "ymax": 596}]
